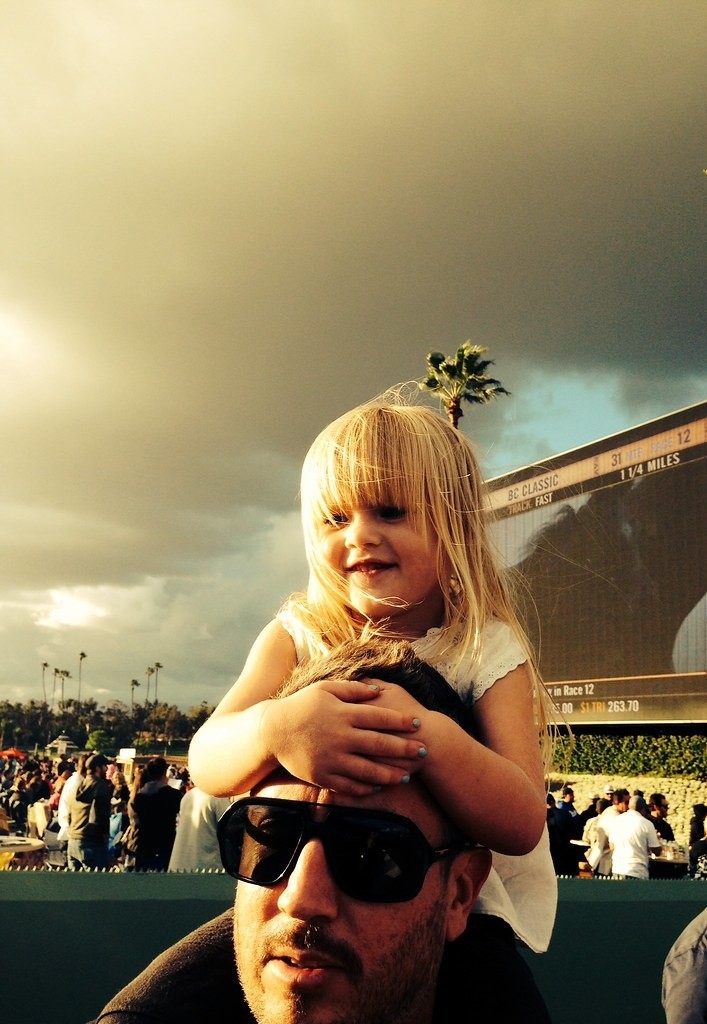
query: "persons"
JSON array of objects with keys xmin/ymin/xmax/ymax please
[
  {"xmin": 85, "ymin": 639, "xmax": 494, "ymax": 1024},
  {"xmin": 0, "ymin": 746, "xmax": 250, "ymax": 874},
  {"xmin": 546, "ymin": 785, "xmax": 707, "ymax": 880},
  {"xmin": 186, "ymin": 381, "xmax": 575, "ymax": 953},
  {"xmin": 661, "ymin": 907, "xmax": 707, "ymax": 1024}
]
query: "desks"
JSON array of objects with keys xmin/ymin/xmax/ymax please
[{"xmin": 0, "ymin": 836, "xmax": 44, "ymax": 852}]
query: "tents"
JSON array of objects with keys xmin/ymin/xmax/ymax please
[{"xmin": 0, "ymin": 748, "xmax": 26, "ymax": 757}]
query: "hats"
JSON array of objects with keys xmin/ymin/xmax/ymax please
[
  {"xmin": 604, "ymin": 784, "xmax": 614, "ymax": 793},
  {"xmin": 85, "ymin": 754, "xmax": 115, "ymax": 769},
  {"xmin": 588, "ymin": 793, "xmax": 601, "ymax": 800}
]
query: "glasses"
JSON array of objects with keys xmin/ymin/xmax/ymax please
[
  {"xmin": 216, "ymin": 797, "xmax": 463, "ymax": 903},
  {"xmin": 662, "ymin": 804, "xmax": 668, "ymax": 809},
  {"xmin": 625, "ymin": 800, "xmax": 629, "ymax": 805}
]
